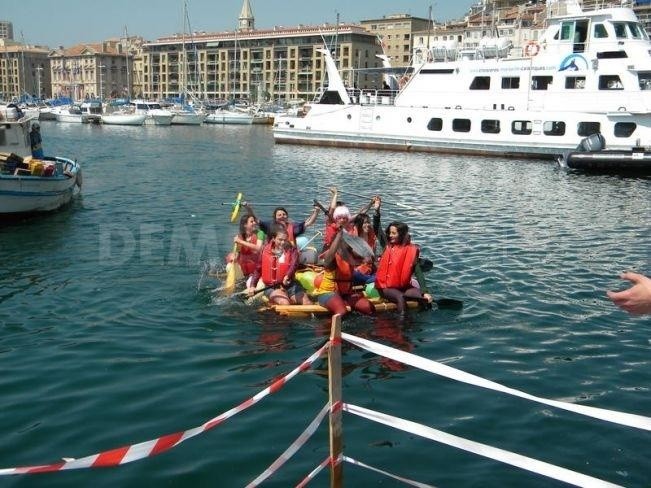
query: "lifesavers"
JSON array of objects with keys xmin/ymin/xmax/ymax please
[{"xmin": 525, "ymin": 42, "xmax": 540, "ymax": 57}]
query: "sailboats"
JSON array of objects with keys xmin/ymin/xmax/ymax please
[{"xmin": 0, "ymin": 0, "xmax": 313, "ymax": 129}]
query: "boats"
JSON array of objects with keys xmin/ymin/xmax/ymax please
[
  {"xmin": 0, "ymin": 151, "xmax": 85, "ymax": 219},
  {"xmin": 209, "ymin": 254, "xmax": 422, "ymax": 316},
  {"xmin": 562, "ymin": 131, "xmax": 651, "ymax": 177},
  {"xmin": 267, "ymin": 0, "xmax": 651, "ymax": 159}
]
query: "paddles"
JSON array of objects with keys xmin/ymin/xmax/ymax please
[
  {"xmin": 232, "ymin": 282, "xmax": 282, "ymax": 304},
  {"xmin": 313, "ymin": 199, "xmax": 374, "ymax": 258},
  {"xmin": 418, "ymin": 258, "xmax": 432, "ymax": 271},
  {"xmin": 226, "ymin": 235, "xmax": 237, "ymax": 292},
  {"xmin": 403, "ymin": 294, "xmax": 464, "ymax": 311},
  {"xmin": 317, "ymin": 184, "xmax": 423, "ymax": 215}
]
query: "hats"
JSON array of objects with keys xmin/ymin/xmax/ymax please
[{"xmin": 332, "ymin": 205, "xmax": 351, "ymax": 223}]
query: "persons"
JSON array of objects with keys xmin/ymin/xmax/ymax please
[
  {"xmin": 30, "ymin": 123, "xmax": 44, "ymax": 158},
  {"xmin": 242, "ymin": 324, "xmax": 419, "ymax": 374},
  {"xmin": 218, "ymin": 187, "xmax": 434, "ymax": 325},
  {"xmin": 608, "ymin": 265, "xmax": 651, "ymax": 318}
]
